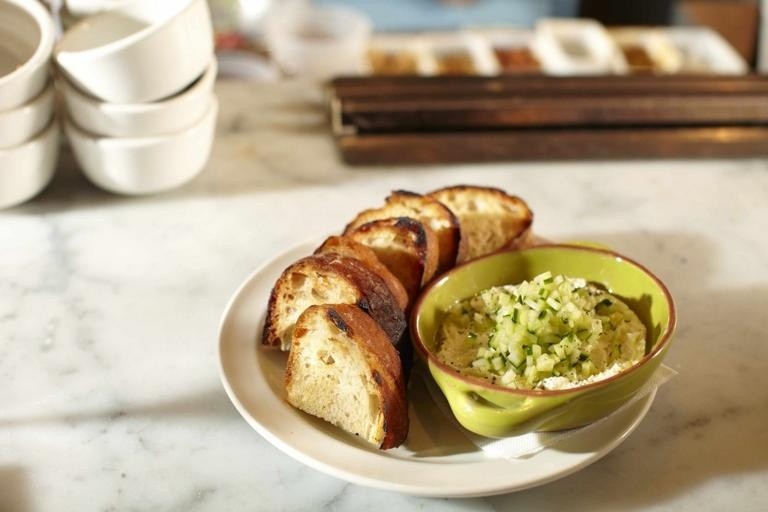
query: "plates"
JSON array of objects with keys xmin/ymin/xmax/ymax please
[
  {"xmin": 367, "ymin": 26, "xmax": 747, "ymax": 81},
  {"xmin": 215, "ymin": 232, "xmax": 664, "ymax": 500}
]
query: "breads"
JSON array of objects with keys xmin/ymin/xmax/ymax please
[
  {"xmin": 283, "ymin": 301, "xmax": 413, "ymax": 453},
  {"xmin": 262, "ymin": 252, "xmax": 413, "ymax": 390},
  {"xmin": 424, "ymin": 183, "xmax": 534, "ymax": 262},
  {"xmin": 352, "ymin": 215, "xmax": 430, "ymax": 302},
  {"xmin": 340, "ymin": 189, "xmax": 462, "ymax": 293},
  {"xmin": 311, "ymin": 235, "xmax": 411, "ymax": 313}
]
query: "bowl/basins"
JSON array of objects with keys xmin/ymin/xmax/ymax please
[
  {"xmin": 2, "ymin": 2, "xmax": 60, "ymax": 209},
  {"xmin": 411, "ymin": 246, "xmax": 676, "ymax": 438},
  {"xmin": 52, "ymin": 0, "xmax": 216, "ymax": 199}
]
